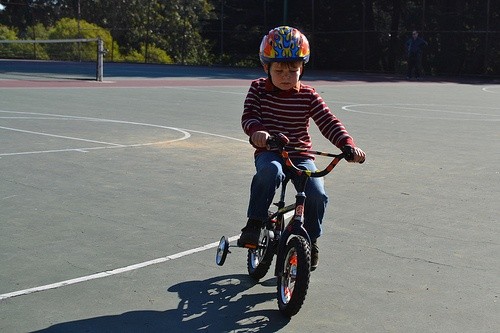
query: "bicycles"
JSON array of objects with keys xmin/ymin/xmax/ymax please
[{"xmin": 216, "ymin": 133, "xmax": 365, "ymax": 317}]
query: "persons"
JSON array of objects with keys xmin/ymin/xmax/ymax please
[{"xmin": 239, "ymin": 26, "xmax": 367, "ymax": 271}]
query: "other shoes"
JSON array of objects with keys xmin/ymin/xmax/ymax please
[
  {"xmin": 312, "ymin": 239, "xmax": 320, "ymax": 269},
  {"xmin": 240, "ymin": 219, "xmax": 261, "ymax": 243}
]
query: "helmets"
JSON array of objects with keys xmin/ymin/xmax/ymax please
[{"xmin": 259, "ymin": 25, "xmax": 311, "ymax": 64}]
